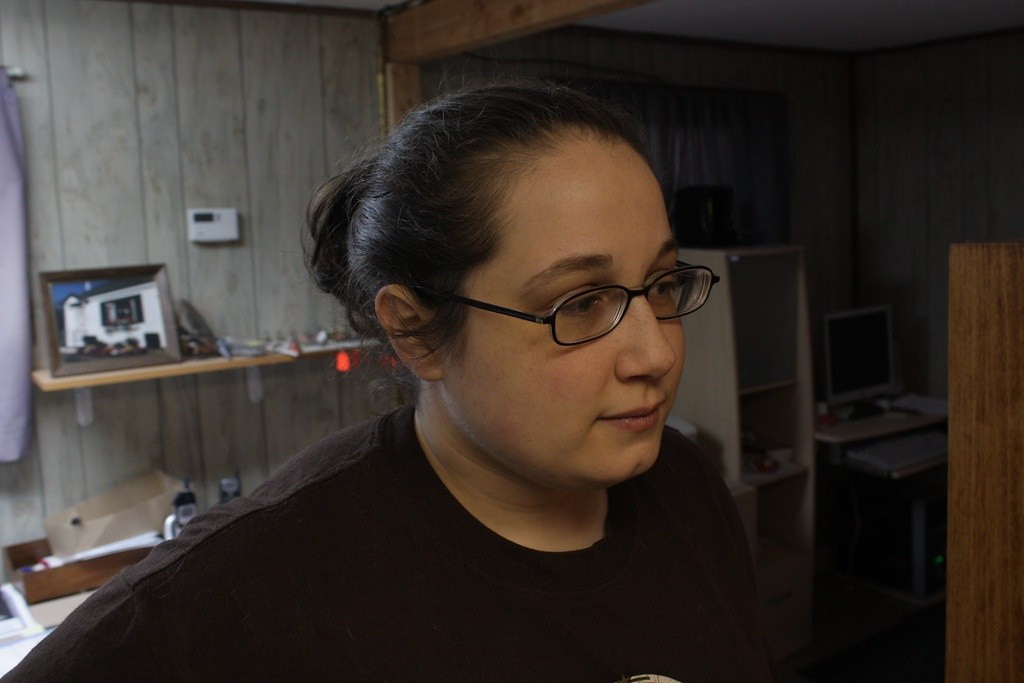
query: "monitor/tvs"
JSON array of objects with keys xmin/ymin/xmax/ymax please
[{"xmin": 825, "ymin": 304, "xmax": 896, "ymax": 417}]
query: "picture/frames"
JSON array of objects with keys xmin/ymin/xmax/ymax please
[{"xmin": 37, "ymin": 262, "xmax": 182, "ymax": 378}]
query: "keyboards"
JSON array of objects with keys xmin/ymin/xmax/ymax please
[{"xmin": 857, "ymin": 430, "xmax": 948, "ymax": 470}]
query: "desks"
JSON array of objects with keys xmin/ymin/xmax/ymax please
[{"xmin": 815, "ymin": 409, "xmax": 940, "ymax": 597}]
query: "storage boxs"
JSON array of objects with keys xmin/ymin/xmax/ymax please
[{"xmin": 2, "ymin": 467, "xmax": 182, "ymax": 606}]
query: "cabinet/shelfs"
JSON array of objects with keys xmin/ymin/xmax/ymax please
[
  {"xmin": 666, "ymin": 245, "xmax": 818, "ymax": 665},
  {"xmin": 946, "ymin": 241, "xmax": 1024, "ymax": 682}
]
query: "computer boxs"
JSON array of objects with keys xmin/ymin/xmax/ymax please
[{"xmin": 831, "ymin": 466, "xmax": 948, "ymax": 594}]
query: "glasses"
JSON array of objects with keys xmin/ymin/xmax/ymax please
[{"xmin": 410, "ymin": 259, "xmax": 720, "ymax": 346}]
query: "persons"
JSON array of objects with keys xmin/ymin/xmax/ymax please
[{"xmin": 0, "ymin": 71, "xmax": 776, "ymax": 683}]
